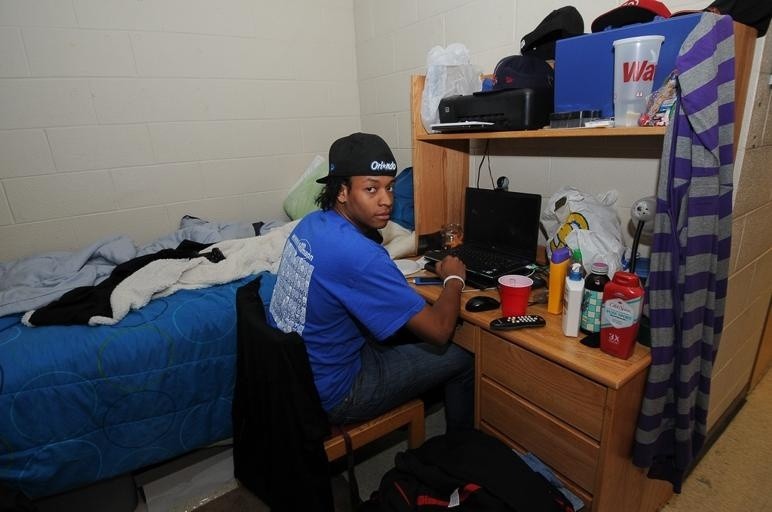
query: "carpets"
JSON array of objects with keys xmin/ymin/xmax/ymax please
[{"xmin": 176, "ymin": 366, "xmax": 772, "ymax": 512}]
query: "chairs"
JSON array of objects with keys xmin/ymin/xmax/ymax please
[{"xmin": 234, "ymin": 284, "xmax": 426, "ymax": 462}]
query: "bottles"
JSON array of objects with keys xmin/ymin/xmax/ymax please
[{"xmin": 580, "ymin": 263, "xmax": 610, "ymax": 334}]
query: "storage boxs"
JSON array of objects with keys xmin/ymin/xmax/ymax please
[{"xmin": 553, "ymin": 12, "xmax": 704, "ymax": 118}]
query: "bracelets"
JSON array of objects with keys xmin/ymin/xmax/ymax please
[{"xmin": 443, "ymin": 273, "xmax": 467, "ymax": 292}]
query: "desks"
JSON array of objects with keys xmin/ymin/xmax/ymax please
[{"xmin": 395, "ymin": 256, "xmax": 655, "ymax": 512}]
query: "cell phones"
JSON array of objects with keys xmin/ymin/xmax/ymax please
[{"xmin": 416, "ymin": 277, "xmax": 443, "ymax": 285}]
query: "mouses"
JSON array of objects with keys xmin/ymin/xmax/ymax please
[{"xmin": 464, "ymin": 295, "xmax": 500, "ymax": 313}]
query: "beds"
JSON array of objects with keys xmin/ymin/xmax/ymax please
[{"xmin": 0, "ymin": 156, "xmax": 414, "ymax": 505}]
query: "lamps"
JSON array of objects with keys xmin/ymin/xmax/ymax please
[{"xmin": 626, "ymin": 194, "xmax": 658, "ymax": 274}]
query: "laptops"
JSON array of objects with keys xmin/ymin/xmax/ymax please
[{"xmin": 423, "ymin": 186, "xmax": 542, "ymax": 286}]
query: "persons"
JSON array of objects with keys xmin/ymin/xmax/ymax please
[{"xmin": 267, "ymin": 132, "xmax": 475, "ymax": 443}]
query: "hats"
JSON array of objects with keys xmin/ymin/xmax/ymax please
[
  {"xmin": 473, "ymin": 57, "xmax": 555, "ymax": 97},
  {"xmin": 522, "ymin": 6, "xmax": 586, "ymax": 56},
  {"xmin": 590, "ymin": 0, "xmax": 671, "ymax": 33},
  {"xmin": 317, "ymin": 132, "xmax": 398, "ymax": 185}
]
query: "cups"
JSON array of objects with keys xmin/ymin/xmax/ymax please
[
  {"xmin": 611, "ymin": 35, "xmax": 664, "ymax": 127},
  {"xmin": 495, "ymin": 273, "xmax": 534, "ymax": 318}
]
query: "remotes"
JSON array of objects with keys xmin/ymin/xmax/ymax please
[{"xmin": 488, "ymin": 312, "xmax": 546, "ymax": 331}]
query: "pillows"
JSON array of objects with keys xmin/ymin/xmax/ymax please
[{"xmin": 282, "ymin": 155, "xmax": 331, "ymax": 223}]
